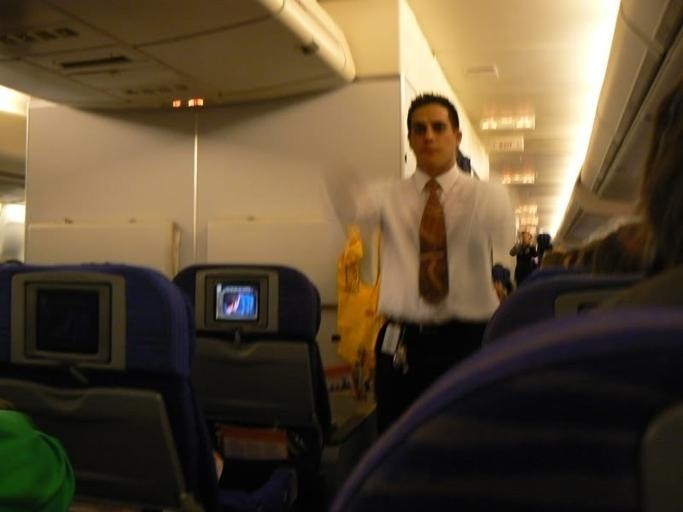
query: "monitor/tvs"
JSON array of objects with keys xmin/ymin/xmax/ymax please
[
  {"xmin": 23, "ymin": 282, "xmax": 111, "ymax": 363},
  {"xmin": 204, "ymin": 276, "xmax": 268, "ymax": 329}
]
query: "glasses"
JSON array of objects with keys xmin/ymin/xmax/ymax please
[{"xmin": 413, "ymin": 122, "xmax": 447, "ymax": 134}]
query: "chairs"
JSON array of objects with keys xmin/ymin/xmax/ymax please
[
  {"xmin": 329, "ymin": 307, "xmax": 683, "ymax": 511},
  {"xmin": 0, "ymin": 261, "xmax": 220, "ymax": 510},
  {"xmin": 480, "ymin": 272, "xmax": 648, "ymax": 346},
  {"xmin": 170, "ymin": 263, "xmax": 378, "ymax": 510}
]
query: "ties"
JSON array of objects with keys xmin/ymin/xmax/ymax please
[{"xmin": 418, "ymin": 180, "xmax": 449, "ymax": 306}]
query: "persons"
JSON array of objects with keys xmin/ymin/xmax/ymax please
[
  {"xmin": 597, "ymin": 82, "xmax": 683, "ymax": 310},
  {"xmin": 510, "ymin": 229, "xmax": 538, "ymax": 286},
  {"xmin": 354, "ymin": 95, "xmax": 517, "ymax": 438},
  {"xmin": 224, "ymin": 293, "xmax": 240, "ymax": 315}
]
